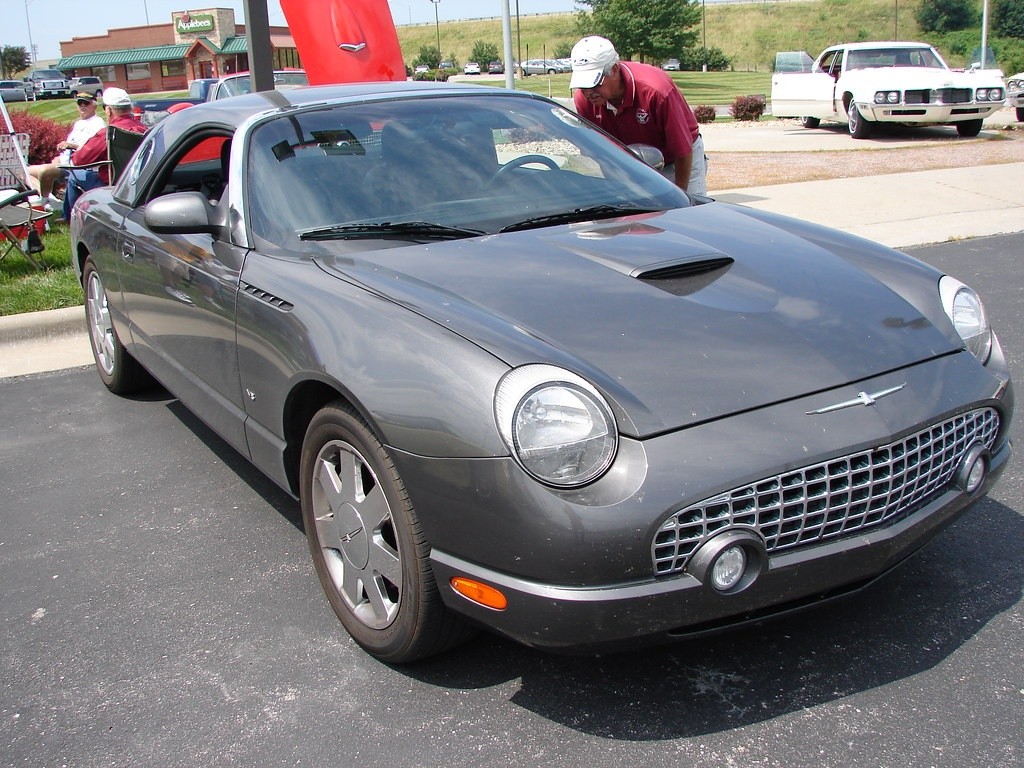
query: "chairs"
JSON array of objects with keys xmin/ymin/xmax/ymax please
[
  {"xmin": 0, "ymin": 124, "xmax": 159, "ymax": 271},
  {"xmin": 894, "ymin": 53, "xmax": 912, "ymax": 66},
  {"xmin": 207, "ymin": 138, "xmax": 333, "ymax": 238},
  {"xmin": 362, "ymin": 122, "xmax": 449, "ymax": 217}
]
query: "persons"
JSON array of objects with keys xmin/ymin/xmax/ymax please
[
  {"xmin": 20, "ymin": 92, "xmax": 106, "ymax": 231},
  {"xmin": 569, "ymin": 36, "xmax": 707, "ymax": 196},
  {"xmin": 55, "ymin": 87, "xmax": 148, "ymax": 223}
]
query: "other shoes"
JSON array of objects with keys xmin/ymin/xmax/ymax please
[
  {"xmin": 53, "ymin": 217, "xmax": 70, "ymax": 225},
  {"xmin": 42, "ymin": 201, "xmax": 54, "ymax": 212}
]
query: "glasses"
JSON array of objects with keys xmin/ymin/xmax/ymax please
[
  {"xmin": 77, "ymin": 100, "xmax": 91, "ymax": 105},
  {"xmin": 581, "ymin": 75, "xmax": 606, "ymax": 90}
]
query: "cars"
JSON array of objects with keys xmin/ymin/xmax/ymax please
[
  {"xmin": 512, "ymin": 58, "xmax": 572, "ymax": 76},
  {"xmin": 1005, "ymin": 71, "xmax": 1024, "ymax": 123},
  {"xmin": 0, "ymin": 68, "xmax": 104, "ymax": 103},
  {"xmin": 771, "ymin": 39, "xmax": 1008, "ymax": 139},
  {"xmin": 463, "ymin": 63, "xmax": 481, "ymax": 76},
  {"xmin": 131, "ymin": 0, "xmax": 408, "ymax": 167},
  {"xmin": 487, "ymin": 61, "xmax": 504, "ymax": 75},
  {"xmin": 661, "ymin": 59, "xmax": 681, "ymax": 71}
]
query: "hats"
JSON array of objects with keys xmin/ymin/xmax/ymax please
[
  {"xmin": 101, "ymin": 87, "xmax": 131, "ymax": 106},
  {"xmin": 76, "ymin": 92, "xmax": 97, "ymax": 103},
  {"xmin": 568, "ymin": 34, "xmax": 620, "ymax": 91}
]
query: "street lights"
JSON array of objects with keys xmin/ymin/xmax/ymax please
[{"xmin": 430, "ymin": 0, "xmax": 443, "ymax": 62}]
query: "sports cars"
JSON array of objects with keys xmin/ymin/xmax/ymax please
[{"xmin": 67, "ymin": 79, "xmax": 1017, "ymax": 668}]
